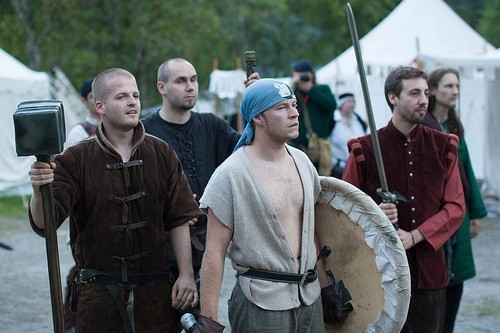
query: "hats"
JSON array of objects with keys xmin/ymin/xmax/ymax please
[
  {"xmin": 81, "ymin": 79, "xmax": 95, "ymax": 97},
  {"xmin": 294, "ymin": 61, "xmax": 312, "ymax": 72},
  {"xmin": 336, "ymin": 93, "xmax": 355, "ymax": 109}
]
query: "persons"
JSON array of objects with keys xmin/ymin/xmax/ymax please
[
  {"xmin": 328, "ymin": 92, "xmax": 368, "ymax": 180},
  {"xmin": 139, "ymin": 57, "xmax": 245, "ymax": 311},
  {"xmin": 26, "ymin": 68, "xmax": 205, "ymax": 333},
  {"xmin": 50, "ymin": 78, "xmax": 102, "ymax": 163},
  {"xmin": 289, "ymin": 62, "xmax": 337, "ymax": 178},
  {"xmin": 427, "ymin": 68, "xmax": 488, "ymax": 333},
  {"xmin": 179, "ymin": 77, "xmax": 347, "ymax": 333},
  {"xmin": 340, "ymin": 66, "xmax": 467, "ymax": 333}
]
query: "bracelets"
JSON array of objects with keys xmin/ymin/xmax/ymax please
[{"xmin": 410, "ymin": 231, "xmax": 415, "ymax": 247}]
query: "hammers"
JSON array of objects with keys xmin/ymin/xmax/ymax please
[{"xmin": 12, "ymin": 99, "xmax": 66, "ymax": 332}]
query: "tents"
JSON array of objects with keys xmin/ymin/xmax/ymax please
[{"xmin": 0, "ymin": 0, "xmax": 500, "ymax": 199}]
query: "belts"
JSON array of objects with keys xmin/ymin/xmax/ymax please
[
  {"xmin": 239, "ymin": 263, "xmax": 318, "ymax": 286},
  {"xmin": 74, "ymin": 268, "xmax": 110, "ymax": 282}
]
